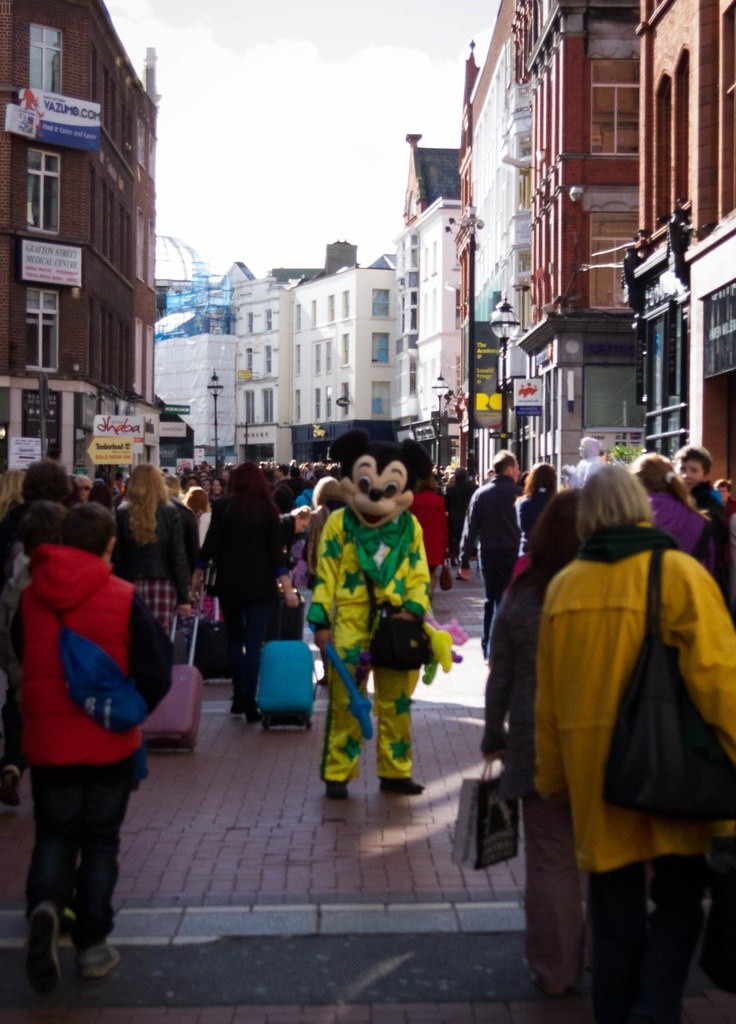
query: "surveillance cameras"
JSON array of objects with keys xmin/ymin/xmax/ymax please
[{"xmin": 569, "ymin": 187, "xmax": 584, "ymax": 201}]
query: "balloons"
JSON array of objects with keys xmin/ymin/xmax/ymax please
[
  {"xmin": 422, "ymin": 612, "xmax": 469, "ymax": 685},
  {"xmin": 326, "ymin": 640, "xmax": 374, "ymax": 741}
]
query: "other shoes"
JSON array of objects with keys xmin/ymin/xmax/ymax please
[
  {"xmin": 0, "ymin": 765, "xmax": 22, "ymax": 807},
  {"xmin": 326, "ymin": 781, "xmax": 348, "ymax": 800},
  {"xmin": 542, "ymin": 977, "xmax": 565, "ymax": 994},
  {"xmin": 380, "ymin": 775, "xmax": 425, "ymax": 794},
  {"xmin": 317, "ymin": 677, "xmax": 327, "ymax": 685}
]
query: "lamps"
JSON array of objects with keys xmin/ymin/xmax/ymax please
[
  {"xmin": 128, "ymin": 395, "xmax": 145, "ymax": 402},
  {"xmin": 106, "ymin": 385, "xmax": 118, "ymax": 392},
  {"xmin": 540, "ymin": 303, "xmax": 557, "ymax": 317},
  {"xmin": 118, "ymin": 389, "xmax": 124, "ymax": 395},
  {"xmin": 88, "ymin": 392, "xmax": 97, "ymax": 400}
]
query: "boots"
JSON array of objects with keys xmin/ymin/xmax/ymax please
[{"xmin": 229, "ymin": 679, "xmax": 262, "ymax": 724}]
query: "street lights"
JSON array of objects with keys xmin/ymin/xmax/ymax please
[
  {"xmin": 208, "ymin": 369, "xmax": 224, "ymax": 468},
  {"xmin": 489, "ymin": 292, "xmax": 521, "ymax": 451},
  {"xmin": 432, "ymin": 372, "xmax": 450, "ymax": 466},
  {"xmin": 446, "ymin": 204, "xmax": 483, "ymax": 479}
]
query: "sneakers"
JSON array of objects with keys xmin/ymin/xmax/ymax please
[
  {"xmin": 25, "ymin": 901, "xmax": 62, "ymax": 996},
  {"xmin": 79, "ymin": 940, "xmax": 120, "ymax": 978}
]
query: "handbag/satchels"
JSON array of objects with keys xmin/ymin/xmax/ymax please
[
  {"xmin": 701, "ymin": 834, "xmax": 736, "ymax": 993},
  {"xmin": 449, "ymin": 756, "xmax": 519, "ymax": 870},
  {"xmin": 603, "ymin": 542, "xmax": 736, "ymax": 821},
  {"xmin": 55, "ymin": 608, "xmax": 149, "ymax": 732},
  {"xmin": 440, "ymin": 555, "xmax": 452, "ymax": 591},
  {"xmin": 202, "ymin": 558, "xmax": 221, "ymax": 594},
  {"xmin": 369, "ymin": 610, "xmax": 427, "ymax": 671}
]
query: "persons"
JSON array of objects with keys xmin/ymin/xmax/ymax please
[
  {"xmin": 459, "ymin": 449, "xmax": 736, "ymax": 998},
  {"xmin": 306, "ymin": 430, "xmax": 434, "ymax": 799},
  {"xmin": 409, "ymin": 467, "xmax": 477, "ymax": 608},
  {"xmin": 9, "ymin": 500, "xmax": 172, "ymax": 995},
  {"xmin": 534, "ymin": 465, "xmax": 736, "ymax": 1024},
  {"xmin": 0, "ymin": 457, "xmax": 344, "ymax": 805}
]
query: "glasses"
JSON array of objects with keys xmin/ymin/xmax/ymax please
[{"xmin": 78, "ymin": 486, "xmax": 90, "ymax": 490}]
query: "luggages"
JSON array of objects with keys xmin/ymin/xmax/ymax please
[
  {"xmin": 138, "ymin": 609, "xmax": 205, "ymax": 755},
  {"xmin": 194, "ymin": 594, "xmax": 233, "ymax": 680},
  {"xmin": 254, "ymin": 593, "xmax": 317, "ymax": 729}
]
